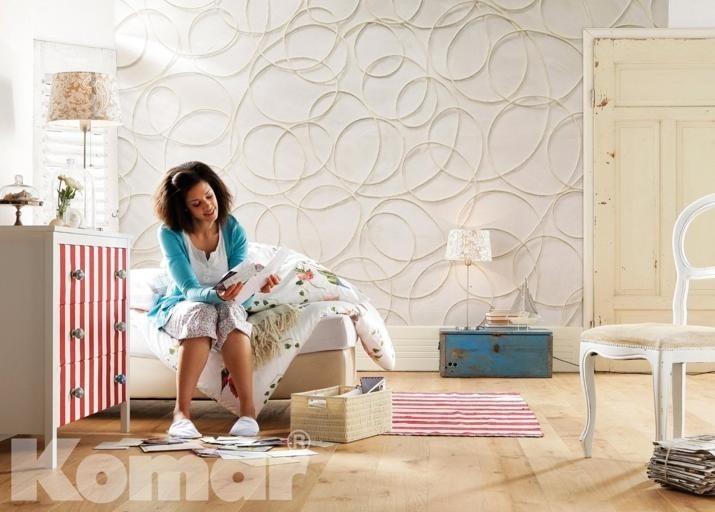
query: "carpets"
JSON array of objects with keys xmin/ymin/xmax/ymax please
[{"xmin": 392, "ymin": 390, "xmax": 545, "ymax": 437}]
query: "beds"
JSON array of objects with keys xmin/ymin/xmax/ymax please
[{"xmin": 130, "ymin": 312, "xmax": 357, "ymax": 399}]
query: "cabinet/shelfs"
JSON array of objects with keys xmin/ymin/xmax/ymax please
[{"xmin": 0, "ymin": 225, "xmax": 134, "ymax": 470}]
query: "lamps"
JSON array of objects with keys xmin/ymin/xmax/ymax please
[
  {"xmin": 47, "ymin": 70, "xmax": 122, "ymax": 232},
  {"xmin": 444, "ymin": 228, "xmax": 493, "ymax": 330}
]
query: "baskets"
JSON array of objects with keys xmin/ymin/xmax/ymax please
[{"xmin": 288, "ymin": 382, "xmax": 394, "ymax": 444}]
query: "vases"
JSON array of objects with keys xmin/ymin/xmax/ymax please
[{"xmin": 49, "ymin": 216, "xmax": 67, "ymax": 225}]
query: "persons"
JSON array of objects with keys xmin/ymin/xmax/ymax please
[{"xmin": 149, "ymin": 159, "xmax": 263, "ymax": 440}]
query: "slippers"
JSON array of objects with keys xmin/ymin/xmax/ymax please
[
  {"xmin": 164, "ymin": 418, "xmax": 203, "ymax": 440},
  {"xmin": 228, "ymin": 415, "xmax": 261, "ymax": 438}
]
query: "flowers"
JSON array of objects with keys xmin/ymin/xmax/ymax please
[{"xmin": 55, "ymin": 175, "xmax": 85, "ymax": 217}]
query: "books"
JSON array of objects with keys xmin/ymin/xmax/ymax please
[
  {"xmin": 92, "ymin": 432, "xmax": 317, "ymax": 461},
  {"xmin": 645, "ymin": 433, "xmax": 715, "ymax": 498},
  {"xmin": 359, "ymin": 374, "xmax": 385, "ymax": 395},
  {"xmin": 480, "ymin": 308, "xmax": 529, "ymax": 331},
  {"xmin": 210, "ymin": 246, "xmax": 298, "ymax": 308}
]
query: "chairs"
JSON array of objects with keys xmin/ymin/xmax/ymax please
[{"xmin": 578, "ymin": 195, "xmax": 715, "ymax": 459}]
